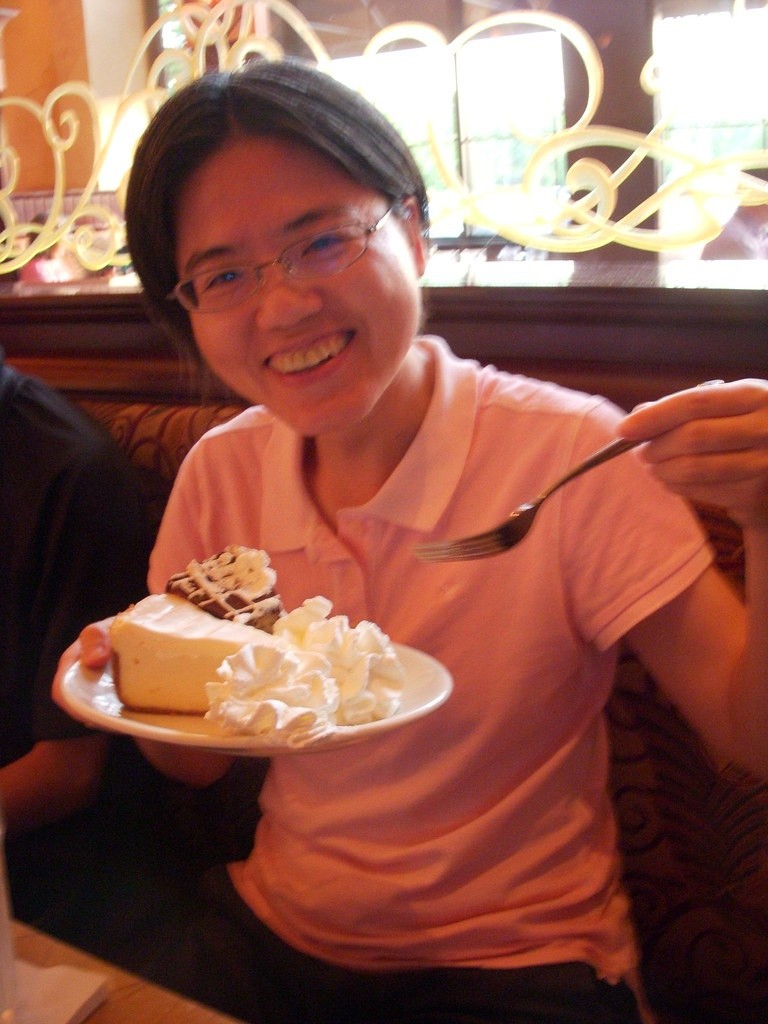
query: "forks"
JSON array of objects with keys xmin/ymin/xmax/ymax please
[{"xmin": 410, "ymin": 378, "xmax": 725, "ymax": 562}]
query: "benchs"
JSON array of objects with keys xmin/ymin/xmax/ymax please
[{"xmin": 0, "ymin": 399, "xmax": 768, "ymax": 1024}]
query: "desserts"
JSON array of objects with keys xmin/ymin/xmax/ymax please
[{"xmin": 105, "ymin": 542, "xmax": 408, "ymax": 750}]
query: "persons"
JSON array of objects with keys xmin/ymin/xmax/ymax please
[
  {"xmin": 51, "ymin": 57, "xmax": 768, "ymax": 1024},
  {"xmin": 0, "ymin": 349, "xmax": 271, "ymax": 953}
]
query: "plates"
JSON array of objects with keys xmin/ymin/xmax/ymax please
[{"xmin": 63, "ymin": 641, "xmax": 454, "ymax": 758}]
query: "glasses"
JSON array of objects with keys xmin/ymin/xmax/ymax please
[{"xmin": 162, "ymin": 199, "xmax": 397, "ymax": 314}]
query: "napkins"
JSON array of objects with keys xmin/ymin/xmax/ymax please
[{"xmin": 13, "ymin": 959, "xmax": 110, "ymax": 1024}]
